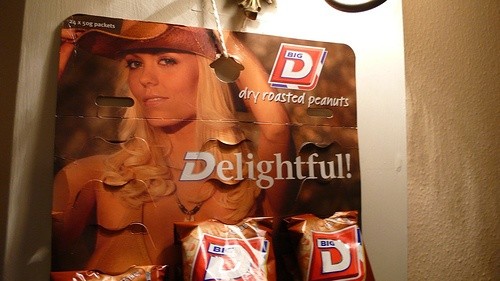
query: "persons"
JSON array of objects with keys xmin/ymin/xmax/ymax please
[{"xmin": 50, "ymin": 23, "xmax": 298, "ymax": 275}]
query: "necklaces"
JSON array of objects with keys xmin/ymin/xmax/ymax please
[{"xmin": 159, "ymin": 157, "xmax": 206, "ymax": 221}]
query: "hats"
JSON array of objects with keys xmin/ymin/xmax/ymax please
[{"xmin": 77, "ymin": 19, "xmax": 219, "ymax": 62}]
